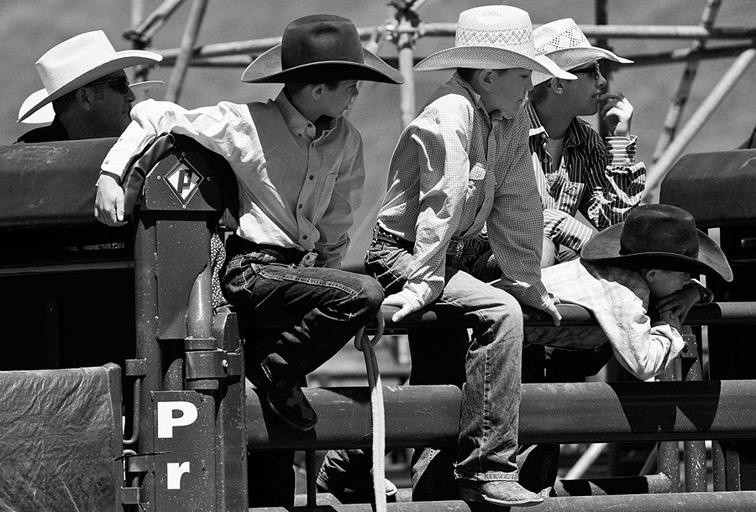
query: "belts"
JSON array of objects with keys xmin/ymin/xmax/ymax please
[
  {"xmin": 373, "ymin": 226, "xmax": 464, "ymax": 261},
  {"xmin": 274, "ymin": 246, "xmax": 318, "ymax": 268}
]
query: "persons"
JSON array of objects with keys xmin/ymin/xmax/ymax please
[
  {"xmin": 505, "ymin": 12, "xmax": 652, "ymax": 268},
  {"xmin": 93, "ymin": 11, "xmax": 406, "ymax": 506},
  {"xmin": 6, "ymin": 24, "xmax": 166, "ymax": 143},
  {"xmin": 316, "ymin": 2, "xmax": 580, "ymax": 508},
  {"xmin": 399, "ymin": 201, "xmax": 743, "ymax": 493}
]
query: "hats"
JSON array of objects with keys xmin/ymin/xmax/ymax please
[
  {"xmin": 579, "ymin": 204, "xmax": 734, "ymax": 283},
  {"xmin": 241, "ymin": 14, "xmax": 406, "ymax": 84},
  {"xmin": 15, "ymin": 29, "xmax": 165, "ymax": 124},
  {"xmin": 411, "ymin": 5, "xmax": 635, "ymax": 86}
]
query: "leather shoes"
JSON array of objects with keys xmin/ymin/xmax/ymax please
[
  {"xmin": 458, "ymin": 478, "xmax": 545, "ymax": 506},
  {"xmin": 260, "ymin": 359, "xmax": 317, "ymax": 431},
  {"xmin": 315, "ymin": 468, "xmax": 397, "ymax": 496}
]
